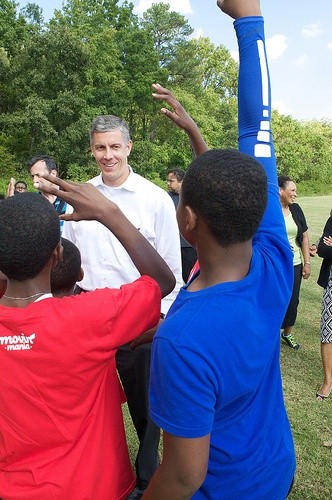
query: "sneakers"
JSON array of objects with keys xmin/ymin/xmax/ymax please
[{"xmin": 281, "ymin": 331, "xmax": 299, "ymax": 350}]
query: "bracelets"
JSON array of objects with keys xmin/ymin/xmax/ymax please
[{"xmin": 304, "ymin": 261, "xmax": 311, "ymax": 265}]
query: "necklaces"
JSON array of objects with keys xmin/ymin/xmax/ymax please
[{"xmin": 2, "ymin": 292, "xmax": 50, "ymax": 301}]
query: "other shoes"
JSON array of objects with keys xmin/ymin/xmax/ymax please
[{"xmin": 316, "ymin": 383, "xmax": 331, "ymax": 400}]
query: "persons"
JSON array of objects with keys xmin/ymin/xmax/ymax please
[
  {"xmin": 141, "ymin": 0, "xmax": 297, "ymax": 500},
  {"xmin": 276, "ymin": 175, "xmax": 332, "ymax": 398},
  {"xmin": 1, "ymin": 157, "xmax": 83, "ymax": 296},
  {"xmin": 164, "ymin": 169, "xmax": 197, "ymax": 284},
  {"xmin": 59, "ymin": 115, "xmax": 182, "ymax": 488},
  {"xmin": 0, "ymin": 171, "xmax": 177, "ymax": 499}
]
